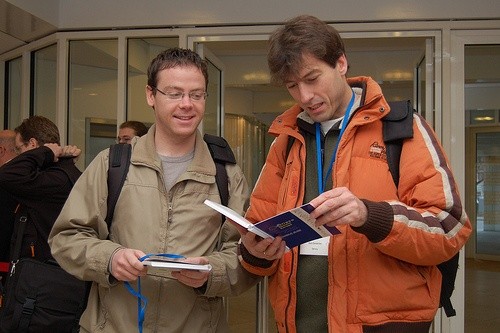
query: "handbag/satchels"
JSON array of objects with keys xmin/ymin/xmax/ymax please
[{"xmin": 2, "ymin": 258, "xmax": 86, "ymax": 333}]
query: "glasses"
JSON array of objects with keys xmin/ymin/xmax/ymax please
[
  {"xmin": 152, "ymin": 86, "xmax": 208, "ymax": 101},
  {"xmin": 14, "ymin": 140, "xmax": 31, "ymax": 153}
]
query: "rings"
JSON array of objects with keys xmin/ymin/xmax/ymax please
[{"xmin": 263, "ymin": 251, "xmax": 271, "ymax": 257}]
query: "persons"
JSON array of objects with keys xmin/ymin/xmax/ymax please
[
  {"xmin": 238, "ymin": 14, "xmax": 473, "ymax": 333},
  {"xmin": 118, "ymin": 121, "xmax": 148, "ymax": 144},
  {"xmin": 47, "ymin": 46, "xmax": 253, "ymax": 333},
  {"xmin": 0, "ymin": 114, "xmax": 93, "ymax": 333}
]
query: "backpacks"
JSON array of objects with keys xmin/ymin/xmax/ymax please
[{"xmin": 285, "ymin": 100, "xmax": 459, "ymax": 307}]
